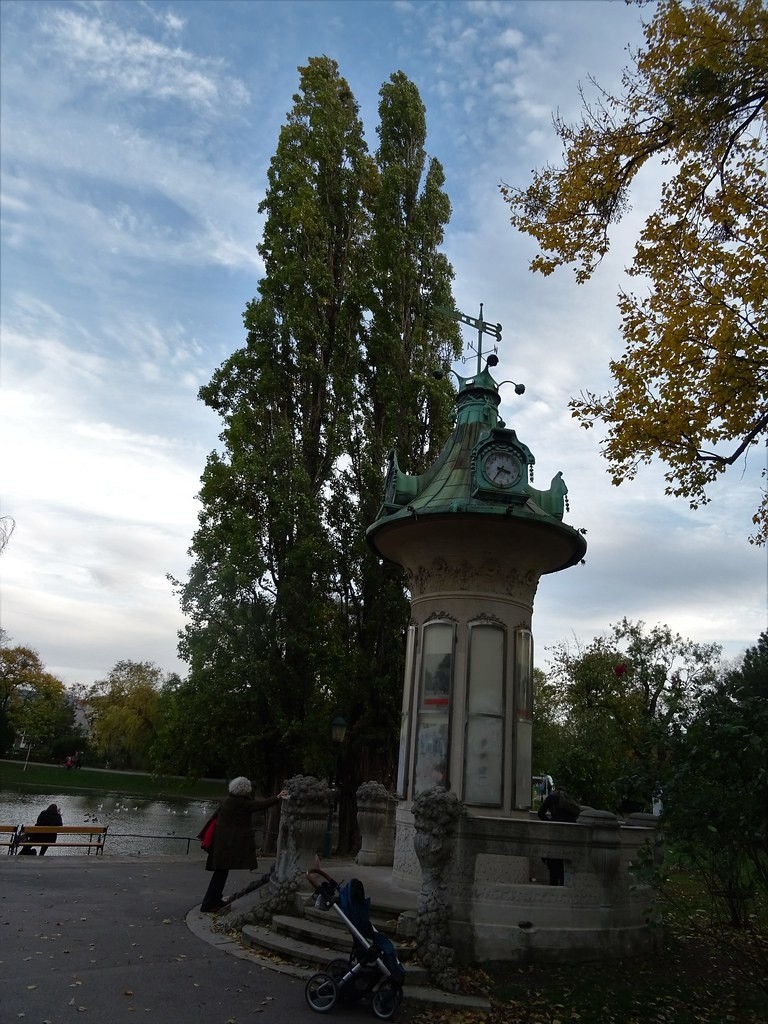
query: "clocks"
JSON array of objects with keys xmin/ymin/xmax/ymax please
[{"xmin": 481, "ymin": 450, "xmax": 522, "ymax": 489}]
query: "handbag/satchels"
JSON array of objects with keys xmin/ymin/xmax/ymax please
[
  {"xmin": 196, "ymin": 807, "xmax": 220, "ymax": 841},
  {"xmin": 201, "ymin": 819, "xmax": 216, "ymax": 853}
]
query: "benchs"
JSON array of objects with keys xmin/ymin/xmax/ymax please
[{"xmin": 0, "ymin": 825, "xmax": 109, "ymax": 857}]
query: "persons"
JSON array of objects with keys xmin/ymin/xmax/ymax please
[
  {"xmin": 537, "ymin": 784, "xmax": 580, "ymax": 886},
  {"xmin": 64, "ymin": 748, "xmax": 84, "ymax": 770},
  {"xmin": 18, "ymin": 804, "xmax": 62, "ymax": 855},
  {"xmin": 333, "ymin": 782, "xmax": 342, "ymax": 812},
  {"xmin": 200, "ymin": 777, "xmax": 289, "ymax": 913},
  {"xmin": 652, "ymin": 781, "xmax": 663, "ymax": 816}
]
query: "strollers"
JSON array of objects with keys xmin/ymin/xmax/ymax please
[{"xmin": 297, "ymin": 866, "xmax": 405, "ymax": 1019}]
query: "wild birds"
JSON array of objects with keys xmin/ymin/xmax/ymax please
[
  {"xmin": 84, "ymin": 812, "xmax": 111, "ymax": 824},
  {"xmin": 166, "ymin": 806, "xmax": 208, "ymax": 815},
  {"xmin": 95, "ymin": 802, "xmax": 138, "ymax": 814}
]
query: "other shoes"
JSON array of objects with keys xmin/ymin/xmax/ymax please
[{"xmin": 201, "ymin": 901, "xmax": 230, "ymax": 912}]
